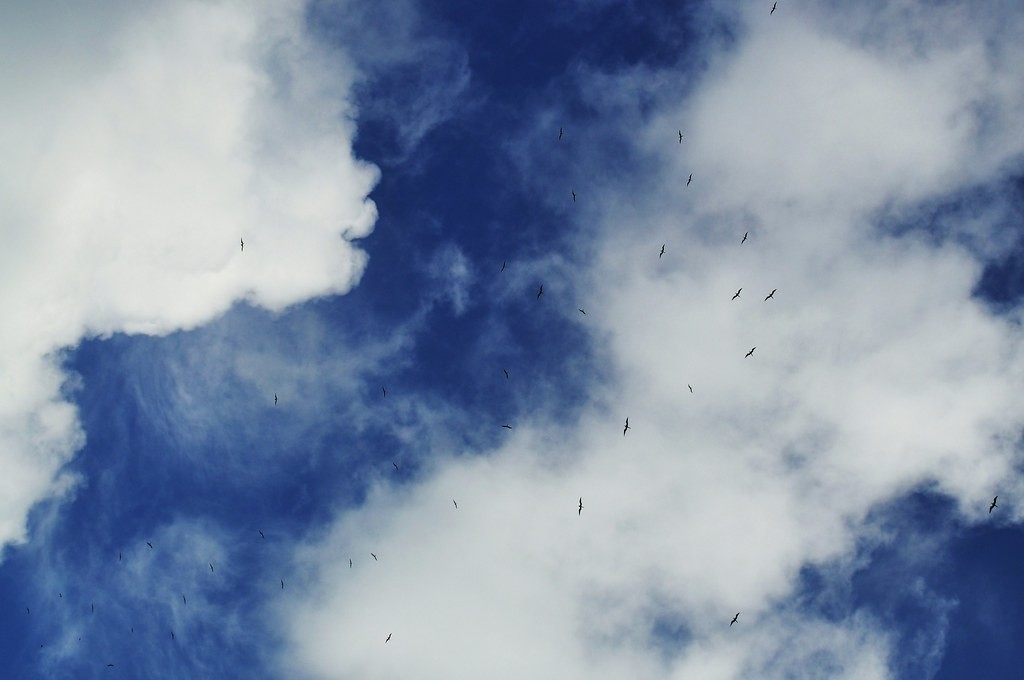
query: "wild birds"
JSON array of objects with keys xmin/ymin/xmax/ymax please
[
  {"xmin": 572, "ymin": 189, "xmax": 576, "ymax": 203},
  {"xmin": 686, "ymin": 173, "xmax": 692, "ymax": 186},
  {"xmin": 27, "ymin": 608, "xmax": 29, "ymax": 614},
  {"xmin": 147, "ymin": 542, "xmax": 153, "ymax": 548},
  {"xmin": 79, "ymin": 638, "xmax": 81, "ymax": 640},
  {"xmin": 732, "ymin": 287, "xmax": 742, "ymax": 300},
  {"xmin": 91, "ymin": 603, "xmax": 94, "ymax": 612},
  {"xmin": 132, "ymin": 627, "xmax": 133, "ymax": 632},
  {"xmin": 559, "ymin": 127, "xmax": 563, "ymax": 140},
  {"xmin": 393, "ymin": 462, "xmax": 399, "ymax": 471},
  {"xmin": 579, "ymin": 308, "xmax": 586, "ymax": 314},
  {"xmin": 576, "ymin": 344, "xmax": 1001, "ymax": 628},
  {"xmin": 504, "ymin": 369, "xmax": 508, "ymax": 379},
  {"xmin": 502, "ymin": 424, "xmax": 512, "ymax": 429},
  {"xmin": 41, "ymin": 645, "xmax": 43, "ymax": 647},
  {"xmin": 536, "ymin": 283, "xmax": 544, "ymax": 300},
  {"xmin": 659, "ymin": 244, "xmax": 666, "ymax": 258},
  {"xmin": 452, "ymin": 499, "xmax": 458, "ymax": 508},
  {"xmin": 171, "ymin": 632, "xmax": 174, "ymax": 639},
  {"xmin": 770, "ymin": 1, "xmax": 777, "ymax": 15},
  {"xmin": 108, "ymin": 664, "xmax": 114, "ymax": 666},
  {"xmin": 383, "ymin": 387, "xmax": 386, "ymax": 397},
  {"xmin": 240, "ymin": 238, "xmax": 244, "ymax": 252},
  {"xmin": 678, "ymin": 129, "xmax": 683, "ymax": 144},
  {"xmin": 275, "ymin": 393, "xmax": 278, "ymax": 405},
  {"xmin": 59, "ymin": 593, "xmax": 62, "ymax": 597},
  {"xmin": 119, "ymin": 552, "xmax": 121, "ymax": 560},
  {"xmin": 741, "ymin": 231, "xmax": 748, "ymax": 244},
  {"xmin": 501, "ymin": 261, "xmax": 505, "ymax": 273},
  {"xmin": 764, "ymin": 288, "xmax": 777, "ymax": 301},
  {"xmin": 182, "ymin": 529, "xmax": 394, "ymax": 644}
]
